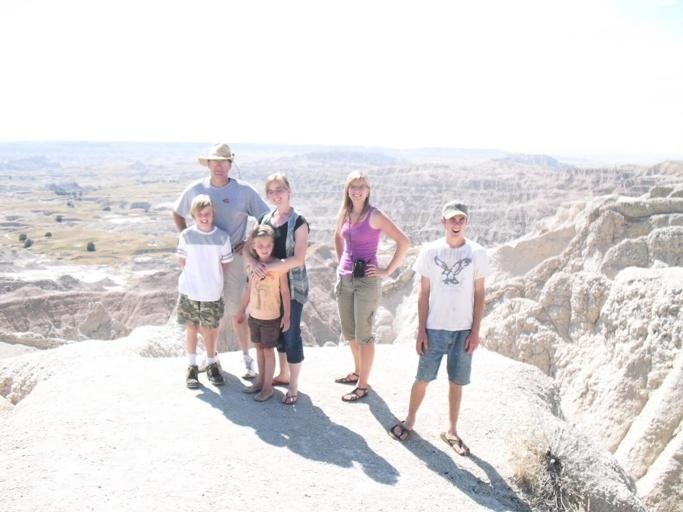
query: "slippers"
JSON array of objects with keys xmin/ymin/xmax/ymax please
[
  {"xmin": 390, "ymin": 421, "xmax": 411, "ymax": 440},
  {"xmin": 441, "ymin": 431, "xmax": 470, "ymax": 456},
  {"xmin": 282, "ymin": 392, "xmax": 298, "ymax": 404},
  {"xmin": 273, "ymin": 377, "xmax": 289, "ymax": 385}
]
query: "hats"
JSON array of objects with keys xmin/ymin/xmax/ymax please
[
  {"xmin": 442, "ymin": 200, "xmax": 468, "ymax": 219},
  {"xmin": 198, "ymin": 143, "xmax": 234, "ymax": 165}
]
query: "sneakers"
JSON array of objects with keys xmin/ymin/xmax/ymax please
[
  {"xmin": 186, "ymin": 365, "xmax": 199, "ymax": 388},
  {"xmin": 207, "ymin": 364, "xmax": 224, "ymax": 384},
  {"xmin": 242, "ymin": 358, "xmax": 255, "ymax": 377}
]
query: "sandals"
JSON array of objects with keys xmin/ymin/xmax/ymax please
[
  {"xmin": 335, "ymin": 372, "xmax": 359, "ymax": 383},
  {"xmin": 254, "ymin": 390, "xmax": 274, "ymax": 401},
  {"xmin": 243, "ymin": 384, "xmax": 262, "ymax": 392},
  {"xmin": 342, "ymin": 388, "xmax": 368, "ymax": 401}
]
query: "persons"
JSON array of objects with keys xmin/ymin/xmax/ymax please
[
  {"xmin": 243, "ymin": 174, "xmax": 308, "ymax": 404},
  {"xmin": 389, "ymin": 201, "xmax": 486, "ymax": 457},
  {"xmin": 175, "ymin": 194, "xmax": 234, "ymax": 388},
  {"xmin": 334, "ymin": 171, "xmax": 409, "ymax": 401},
  {"xmin": 235, "ymin": 225, "xmax": 291, "ymax": 402},
  {"xmin": 173, "ymin": 143, "xmax": 272, "ymax": 379}
]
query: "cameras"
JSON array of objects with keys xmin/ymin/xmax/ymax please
[{"xmin": 352, "ymin": 258, "xmax": 365, "ymax": 279}]
